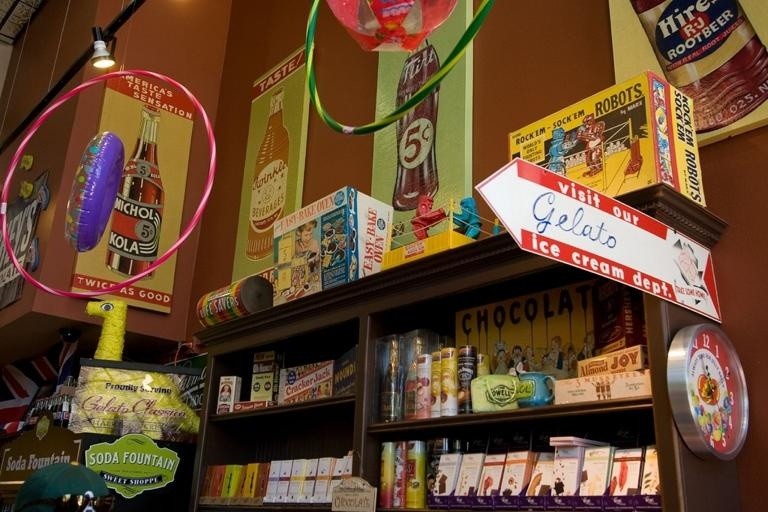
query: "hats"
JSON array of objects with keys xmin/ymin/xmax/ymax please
[{"xmin": 16, "ymin": 493, "xmax": 118, "ymax": 512}]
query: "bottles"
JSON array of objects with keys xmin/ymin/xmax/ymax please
[
  {"xmin": 380, "ymin": 336, "xmax": 492, "ymax": 508},
  {"xmin": 104, "ymin": 104, "xmax": 166, "ymax": 281},
  {"xmin": 392, "ymin": 37, "xmax": 441, "ymax": 213},
  {"xmin": 28, "ymin": 375, "xmax": 76, "ymax": 431},
  {"xmin": 245, "ymin": 86, "xmax": 290, "ymax": 263},
  {"xmin": 628, "ymin": 0, "xmax": 768, "ymax": 132}
]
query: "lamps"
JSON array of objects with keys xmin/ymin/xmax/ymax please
[{"xmin": 91, "ymin": 25, "xmax": 117, "ymax": 70}]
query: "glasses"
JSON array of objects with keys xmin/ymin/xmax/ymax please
[{"xmin": 11, "ymin": 462, "xmax": 111, "ymax": 512}]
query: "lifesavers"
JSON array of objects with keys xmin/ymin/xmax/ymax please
[{"xmin": 64, "ymin": 130, "xmax": 124, "ymax": 253}]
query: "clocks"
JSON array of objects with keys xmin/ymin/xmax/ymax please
[{"xmin": 666, "ymin": 322, "xmax": 750, "ymax": 463}]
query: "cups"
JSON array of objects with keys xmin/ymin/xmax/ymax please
[{"xmin": 516, "ymin": 371, "xmax": 556, "ymax": 408}]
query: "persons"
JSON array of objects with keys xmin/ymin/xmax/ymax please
[
  {"xmin": 293, "ymin": 219, "xmax": 318, "ymax": 252},
  {"xmin": 494, "ymin": 335, "xmax": 578, "ymax": 376}
]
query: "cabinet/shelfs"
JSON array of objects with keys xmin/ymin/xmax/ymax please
[{"xmin": 185, "ymin": 184, "xmax": 742, "ymax": 510}]
